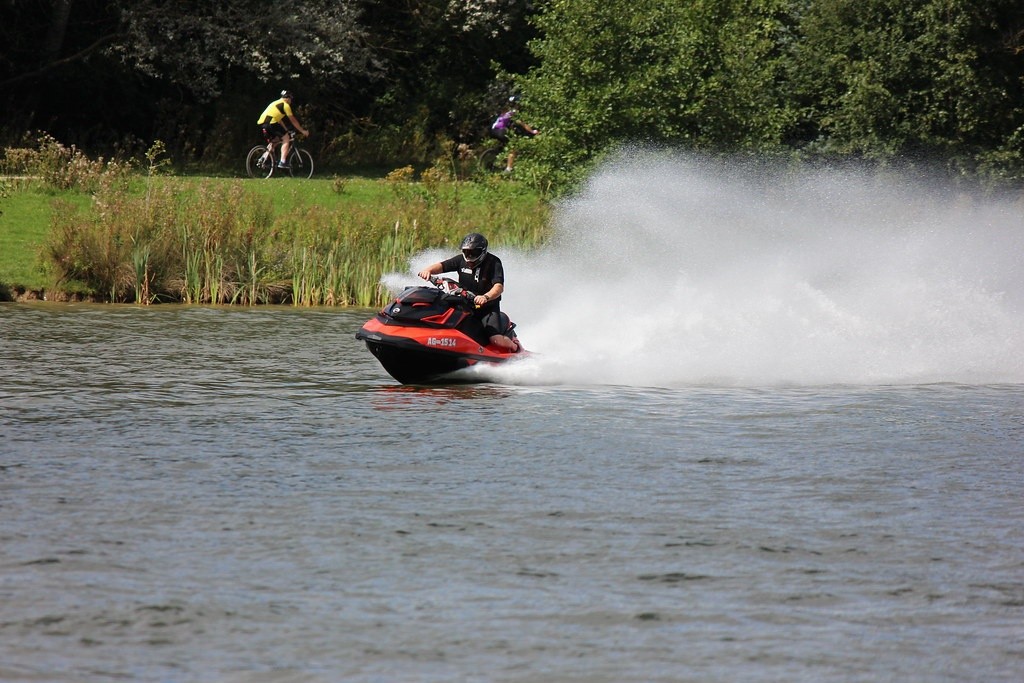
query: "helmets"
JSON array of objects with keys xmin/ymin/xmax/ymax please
[
  {"xmin": 461, "ymin": 233, "xmax": 488, "ymax": 269},
  {"xmin": 508, "ymin": 96, "xmax": 519, "ymax": 103},
  {"xmin": 281, "ymin": 90, "xmax": 294, "ymax": 98}
]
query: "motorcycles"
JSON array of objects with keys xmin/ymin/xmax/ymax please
[{"xmin": 355, "ymin": 272, "xmax": 533, "ymax": 385}]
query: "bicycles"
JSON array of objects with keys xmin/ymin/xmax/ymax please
[
  {"xmin": 480, "ymin": 133, "xmax": 535, "ymax": 173},
  {"xmin": 245, "ymin": 129, "xmax": 315, "ymax": 180}
]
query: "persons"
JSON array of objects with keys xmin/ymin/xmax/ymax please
[
  {"xmin": 487, "ymin": 93, "xmax": 542, "ymax": 173},
  {"xmin": 417, "ymin": 232, "xmax": 522, "ymax": 353},
  {"xmin": 257, "ymin": 89, "xmax": 310, "ymax": 169}
]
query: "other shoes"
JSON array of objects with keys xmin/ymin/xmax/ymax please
[
  {"xmin": 504, "ymin": 168, "xmax": 514, "ymax": 174},
  {"xmin": 278, "ymin": 162, "xmax": 290, "ymax": 169},
  {"xmin": 259, "ymin": 157, "xmax": 269, "ymax": 166}
]
